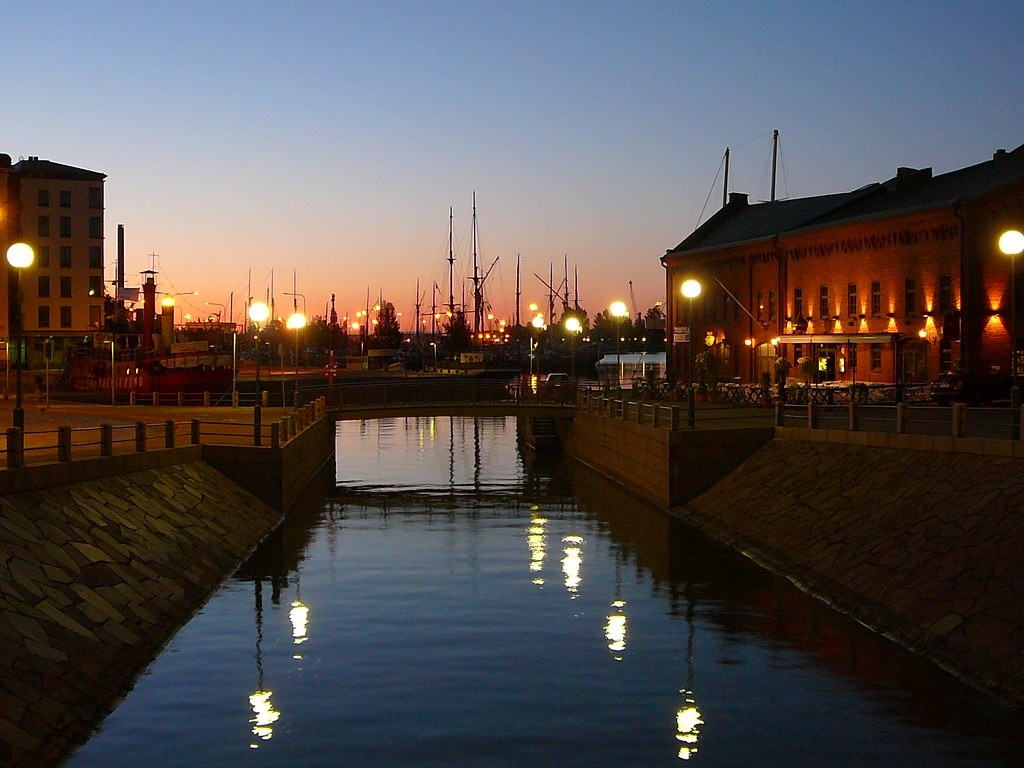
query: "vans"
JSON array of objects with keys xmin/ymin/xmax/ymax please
[{"xmin": 546, "ymin": 373, "xmax": 569, "ymax": 389}]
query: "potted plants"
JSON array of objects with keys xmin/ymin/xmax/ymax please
[
  {"xmin": 642, "ymin": 351, "xmax": 721, "ymax": 403},
  {"xmin": 761, "ymin": 364, "xmax": 786, "ymax": 409}
]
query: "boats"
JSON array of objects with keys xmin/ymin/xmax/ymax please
[{"xmin": 65, "ymin": 270, "xmax": 242, "ymax": 403}]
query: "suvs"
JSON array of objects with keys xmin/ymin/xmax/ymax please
[{"xmin": 930, "ymin": 368, "xmax": 1016, "ymax": 405}]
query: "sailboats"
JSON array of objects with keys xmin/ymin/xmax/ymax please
[{"xmin": 417, "ymin": 191, "xmax": 522, "ymax": 380}]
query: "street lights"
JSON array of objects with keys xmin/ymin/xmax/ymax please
[
  {"xmin": 102, "ymin": 339, "xmax": 115, "ymax": 405},
  {"xmin": 999, "ymin": 230, "xmax": 1024, "ymax": 438},
  {"xmin": 565, "ymin": 317, "xmax": 580, "ymax": 396},
  {"xmin": 289, "ymin": 313, "xmax": 305, "ymax": 411},
  {"xmin": 250, "ymin": 302, "xmax": 268, "ymax": 406},
  {"xmin": 682, "ymin": 280, "xmax": 701, "ymax": 428},
  {"xmin": 609, "ymin": 301, "xmax": 627, "ymax": 416},
  {"xmin": 6, "ymin": 242, "xmax": 34, "ymax": 457}
]
